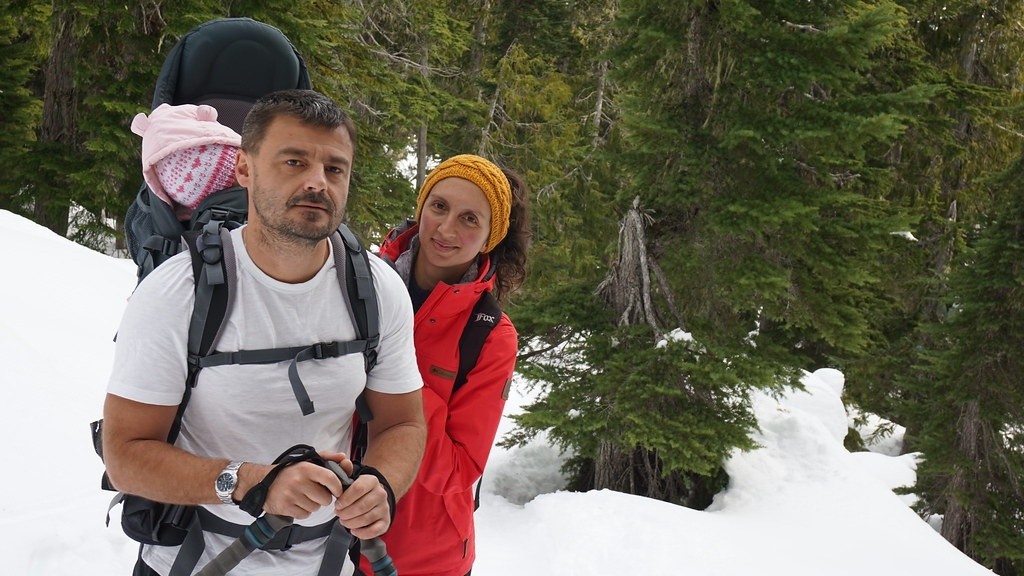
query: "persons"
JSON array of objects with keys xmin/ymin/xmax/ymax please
[
  {"xmin": 352, "ymin": 153, "xmax": 529, "ymax": 576},
  {"xmin": 103, "ymin": 89, "xmax": 426, "ymax": 576},
  {"xmin": 132, "ymin": 104, "xmax": 244, "ymax": 226}
]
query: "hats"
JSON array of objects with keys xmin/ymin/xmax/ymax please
[
  {"xmin": 153, "ymin": 145, "xmax": 237, "ymax": 211},
  {"xmin": 416, "ymin": 154, "xmax": 512, "ymax": 254}
]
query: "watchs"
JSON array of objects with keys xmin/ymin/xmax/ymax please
[{"xmin": 215, "ymin": 460, "xmax": 242, "ymax": 505}]
